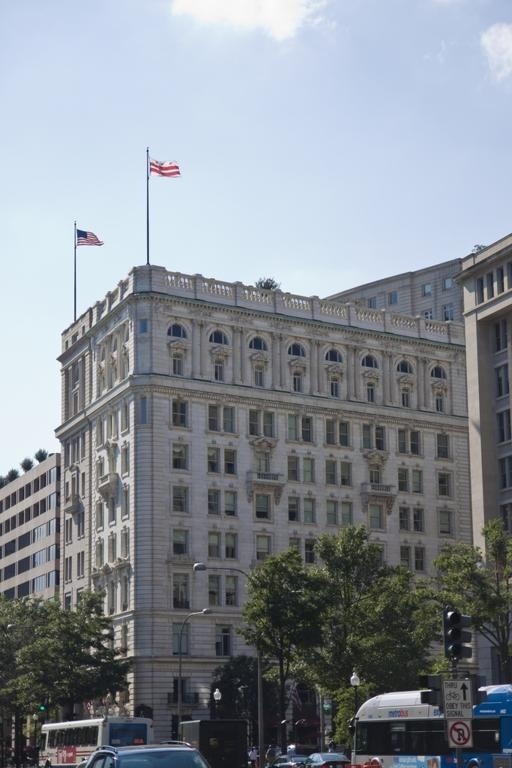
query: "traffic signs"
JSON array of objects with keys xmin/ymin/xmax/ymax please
[{"xmin": 440, "ymin": 680, "xmax": 474, "ymax": 721}]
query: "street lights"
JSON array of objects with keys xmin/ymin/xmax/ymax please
[
  {"xmin": 212, "ymin": 685, "xmax": 224, "ymax": 718},
  {"xmin": 189, "ymin": 561, "xmax": 269, "ymax": 748},
  {"xmin": 177, "ymin": 607, "xmax": 214, "ymax": 743},
  {"xmin": 5, "ymin": 623, "xmax": 33, "ymax": 630},
  {"xmin": 349, "ymin": 671, "xmax": 361, "ymax": 712},
  {"xmin": 280, "ymin": 718, "xmax": 311, "ymax": 756}
]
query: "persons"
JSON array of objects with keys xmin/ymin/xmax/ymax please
[
  {"xmin": 264, "ymin": 744, "xmax": 275, "ymax": 768},
  {"xmin": 328, "ymin": 740, "xmax": 336, "ymax": 752},
  {"xmin": 248, "ymin": 746, "xmax": 257, "ymax": 768}
]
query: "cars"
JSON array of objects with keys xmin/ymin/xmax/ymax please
[{"xmin": 249, "ymin": 745, "xmax": 351, "ymax": 767}]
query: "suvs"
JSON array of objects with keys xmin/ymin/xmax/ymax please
[{"xmin": 86, "ymin": 737, "xmax": 216, "ymax": 768}]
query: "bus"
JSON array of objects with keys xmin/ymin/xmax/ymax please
[
  {"xmin": 347, "ymin": 682, "xmax": 511, "ymax": 768},
  {"xmin": 36, "ymin": 715, "xmax": 160, "ymax": 768}
]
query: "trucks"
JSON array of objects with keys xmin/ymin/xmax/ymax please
[{"xmin": 173, "ymin": 717, "xmax": 250, "ymax": 768}]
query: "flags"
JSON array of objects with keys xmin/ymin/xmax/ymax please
[
  {"xmin": 149, "ymin": 158, "xmax": 182, "ymax": 178},
  {"xmin": 76, "ymin": 229, "xmax": 104, "ymax": 246}
]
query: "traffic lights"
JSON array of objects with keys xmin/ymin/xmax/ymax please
[
  {"xmin": 457, "ymin": 608, "xmax": 472, "ymax": 660},
  {"xmin": 442, "ymin": 605, "xmax": 462, "ymax": 660}
]
